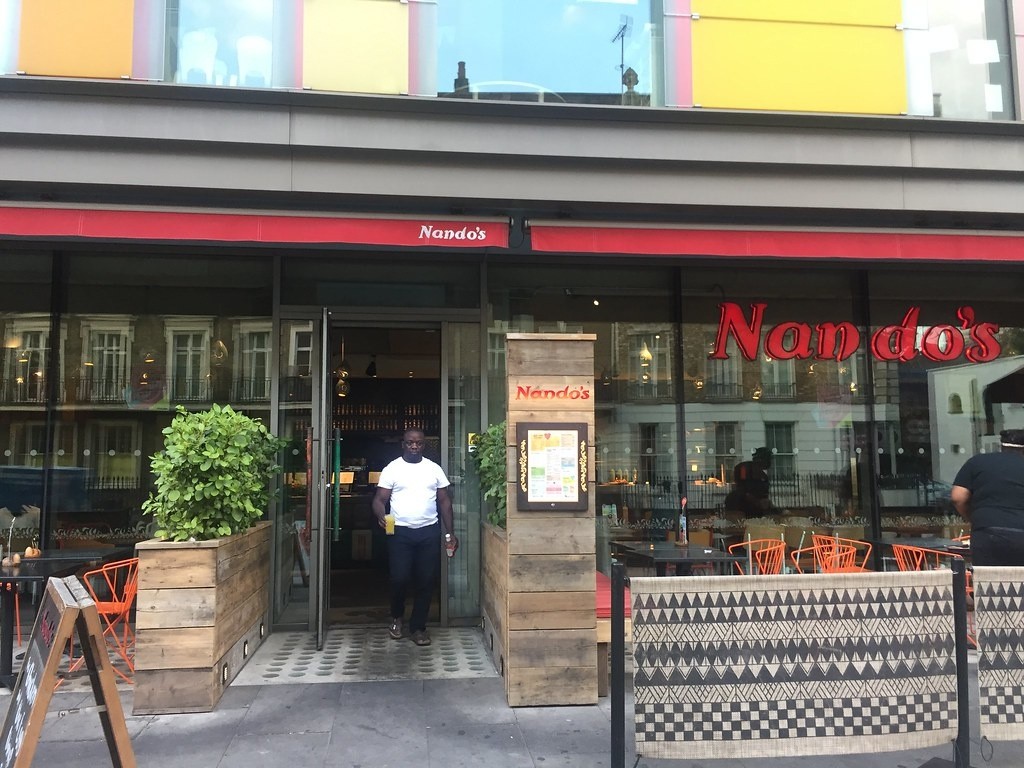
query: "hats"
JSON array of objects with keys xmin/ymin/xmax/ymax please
[
  {"xmin": 999, "ymin": 430, "xmax": 1023, "ymax": 448},
  {"xmin": 753, "ymin": 447, "xmax": 774, "ymax": 461}
]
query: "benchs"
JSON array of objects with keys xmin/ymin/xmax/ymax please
[{"xmin": 594, "ymin": 569, "xmax": 633, "ymax": 698}]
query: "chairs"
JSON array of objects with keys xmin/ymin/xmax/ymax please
[
  {"xmin": 52, "ymin": 557, "xmax": 138, "ymax": 690},
  {"xmin": 665, "ymin": 525, "xmax": 978, "ymax": 649}
]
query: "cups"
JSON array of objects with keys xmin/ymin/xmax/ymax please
[{"xmin": 383, "ymin": 514, "xmax": 395, "ymax": 536}]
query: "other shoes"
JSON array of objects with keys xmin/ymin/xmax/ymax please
[
  {"xmin": 409, "ymin": 630, "xmax": 431, "ymax": 646},
  {"xmin": 389, "ymin": 618, "xmax": 401, "ymax": 639}
]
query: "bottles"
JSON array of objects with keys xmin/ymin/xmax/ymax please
[
  {"xmin": 609, "ymin": 467, "xmax": 637, "ymax": 484},
  {"xmin": 445, "ymin": 534, "xmax": 454, "ymax": 558}
]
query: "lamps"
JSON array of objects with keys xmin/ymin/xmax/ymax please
[
  {"xmin": 748, "ymin": 383, "xmax": 763, "ymax": 400},
  {"xmin": 639, "ymin": 368, "xmax": 651, "ymax": 385},
  {"xmin": 638, "ymin": 341, "xmax": 653, "ymax": 366}
]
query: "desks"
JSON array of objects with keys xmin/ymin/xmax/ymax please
[
  {"xmin": 611, "ymin": 540, "xmax": 750, "ymax": 577},
  {"xmin": 21, "ymin": 547, "xmax": 135, "ymax": 659},
  {"xmin": 0, "ymin": 560, "xmax": 90, "ymax": 687},
  {"xmin": 858, "ymin": 536, "xmax": 969, "ymax": 570}
]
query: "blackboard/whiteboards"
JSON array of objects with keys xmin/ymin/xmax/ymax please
[{"xmin": 0, "ymin": 575, "xmax": 80, "ymax": 768}]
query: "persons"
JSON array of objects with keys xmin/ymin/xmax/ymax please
[
  {"xmin": 724, "ymin": 446, "xmax": 773, "ymax": 516},
  {"xmin": 952, "ymin": 429, "xmax": 1024, "ymax": 567},
  {"xmin": 371, "ymin": 426, "xmax": 459, "ymax": 646},
  {"xmin": 0, "ymin": 485, "xmax": 42, "ymax": 598}
]
